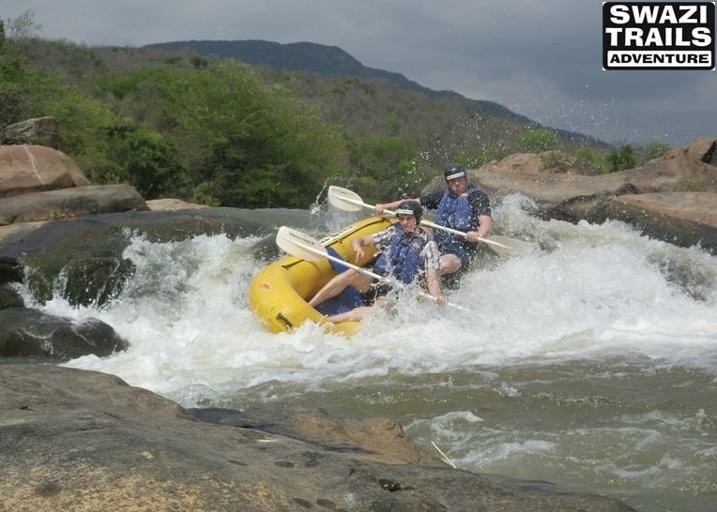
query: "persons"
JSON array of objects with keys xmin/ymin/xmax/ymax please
[
  {"xmin": 375, "ymin": 163, "xmax": 492, "ymax": 275},
  {"xmin": 308, "ymin": 201, "xmax": 447, "ymax": 324}
]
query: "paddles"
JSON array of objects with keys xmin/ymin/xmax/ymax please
[
  {"xmin": 275, "ymin": 226, "xmax": 472, "ymax": 312},
  {"xmin": 328, "ymin": 185, "xmax": 513, "ymax": 248}
]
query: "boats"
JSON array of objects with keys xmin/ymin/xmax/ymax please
[{"xmin": 246, "ymin": 213, "xmax": 407, "ymax": 341}]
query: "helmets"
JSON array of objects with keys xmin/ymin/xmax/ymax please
[
  {"xmin": 395, "ymin": 201, "xmax": 424, "ymax": 225},
  {"xmin": 444, "ymin": 165, "xmax": 466, "ymax": 182}
]
